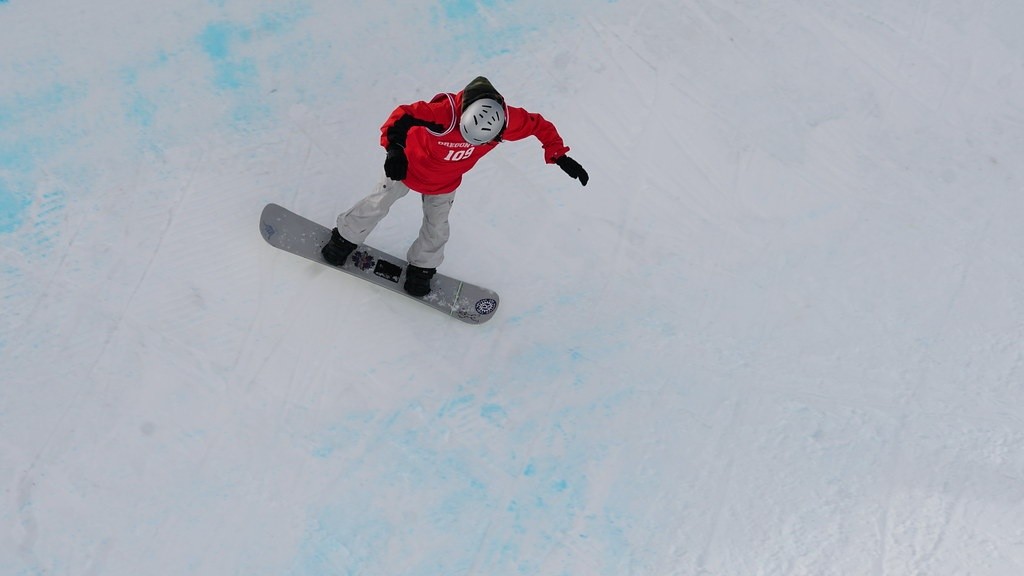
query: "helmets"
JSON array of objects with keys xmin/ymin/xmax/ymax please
[{"xmin": 460, "ymin": 98, "xmax": 504, "ymax": 145}]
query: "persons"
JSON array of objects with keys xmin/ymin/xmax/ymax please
[{"xmin": 321, "ymin": 76, "xmax": 589, "ymax": 298}]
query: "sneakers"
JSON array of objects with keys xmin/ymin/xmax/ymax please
[
  {"xmin": 404, "ymin": 263, "xmax": 437, "ymax": 297},
  {"xmin": 321, "ymin": 227, "xmax": 358, "ymax": 266}
]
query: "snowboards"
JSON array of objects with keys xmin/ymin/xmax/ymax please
[{"xmin": 259, "ymin": 202, "xmax": 500, "ymax": 326}]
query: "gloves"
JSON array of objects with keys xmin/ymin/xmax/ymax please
[
  {"xmin": 384, "ymin": 142, "xmax": 409, "ymax": 181},
  {"xmin": 552, "ymin": 154, "xmax": 589, "ymax": 187}
]
